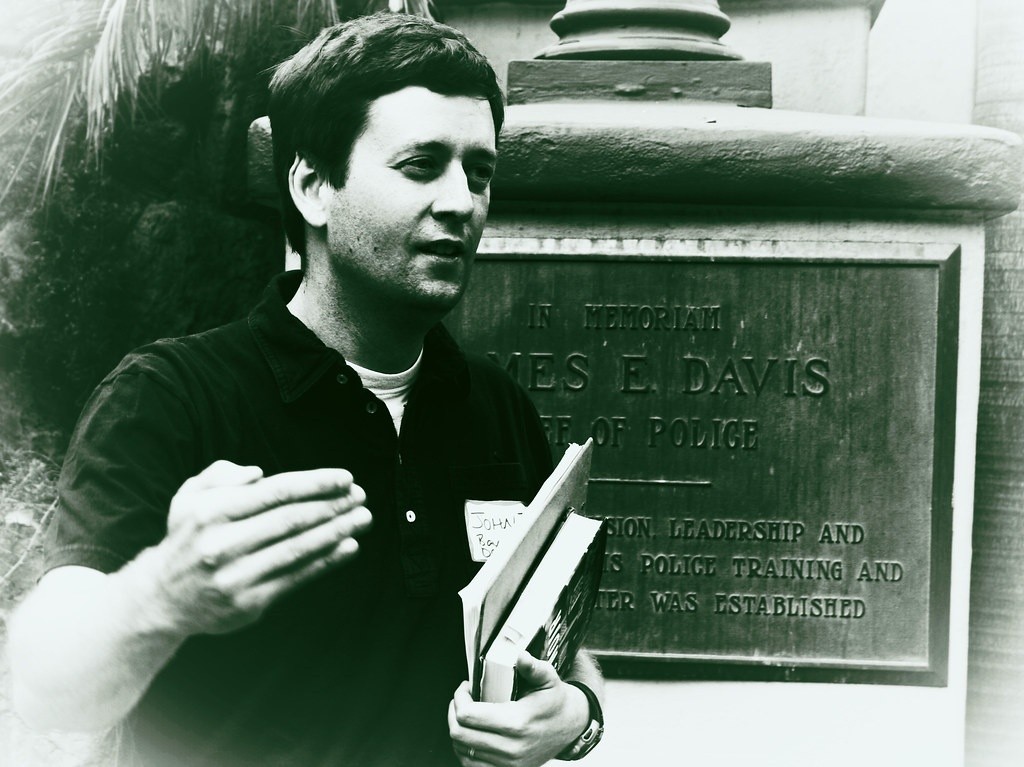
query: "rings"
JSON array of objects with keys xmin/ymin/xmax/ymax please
[{"xmin": 467, "ymin": 747, "xmax": 475, "ymax": 761}]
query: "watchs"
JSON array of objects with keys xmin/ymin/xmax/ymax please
[{"xmin": 553, "ymin": 680, "xmax": 604, "ymax": 762}]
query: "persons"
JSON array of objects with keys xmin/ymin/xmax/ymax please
[{"xmin": 6, "ymin": 12, "xmax": 605, "ymax": 767}]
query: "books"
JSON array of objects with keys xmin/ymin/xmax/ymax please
[{"xmin": 458, "ymin": 435, "xmax": 603, "ymax": 705}]
